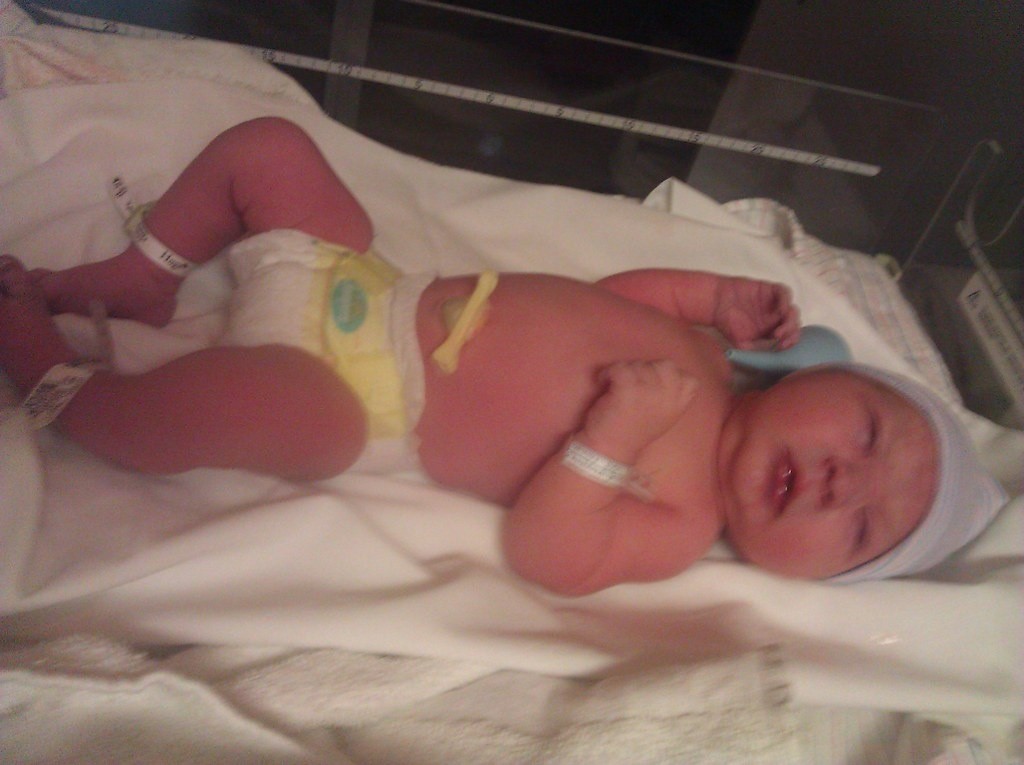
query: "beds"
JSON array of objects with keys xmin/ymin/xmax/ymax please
[{"xmin": 1, "ymin": 2, "xmax": 1024, "ymax": 765}]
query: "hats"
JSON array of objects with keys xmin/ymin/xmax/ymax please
[{"xmin": 776, "ymin": 363, "xmax": 1011, "ymax": 584}]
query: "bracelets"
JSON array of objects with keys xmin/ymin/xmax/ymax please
[{"xmin": 565, "ymin": 440, "xmax": 632, "ymax": 488}]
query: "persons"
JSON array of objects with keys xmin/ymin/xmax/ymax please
[{"xmin": 0, "ymin": 115, "xmax": 1011, "ymax": 597}]
